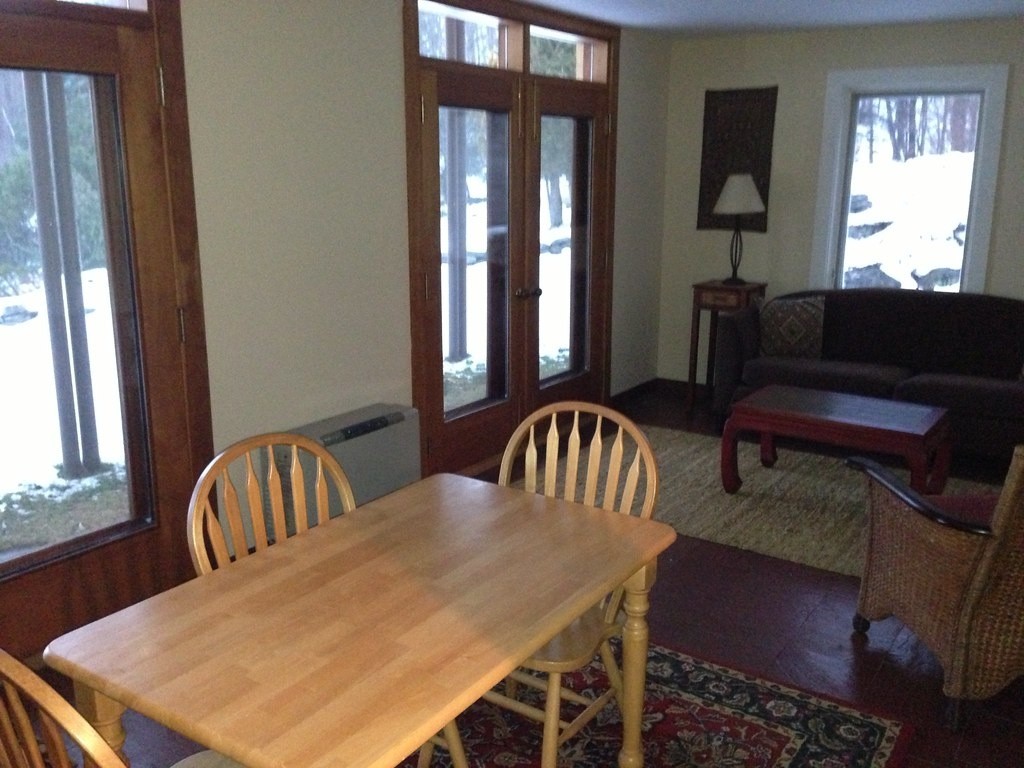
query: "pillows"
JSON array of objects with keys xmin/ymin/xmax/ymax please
[{"xmin": 760, "ymin": 297, "xmax": 824, "ymax": 359}]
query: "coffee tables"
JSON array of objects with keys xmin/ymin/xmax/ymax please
[{"xmin": 720, "ymin": 385, "xmax": 952, "ymax": 496}]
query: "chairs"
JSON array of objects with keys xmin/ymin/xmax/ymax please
[
  {"xmin": 846, "ymin": 446, "xmax": 1024, "ymax": 734},
  {"xmin": 187, "ymin": 432, "xmax": 466, "ymax": 768},
  {"xmin": 0, "ymin": 652, "xmax": 252, "ymax": 768},
  {"xmin": 484, "ymin": 402, "xmax": 655, "ymax": 768}
]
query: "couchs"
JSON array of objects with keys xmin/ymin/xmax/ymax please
[{"xmin": 729, "ymin": 289, "xmax": 1024, "ymax": 453}]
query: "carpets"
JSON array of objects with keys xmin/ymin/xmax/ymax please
[
  {"xmin": 401, "ymin": 637, "xmax": 914, "ymax": 768},
  {"xmin": 510, "ymin": 424, "xmax": 1002, "ymax": 578}
]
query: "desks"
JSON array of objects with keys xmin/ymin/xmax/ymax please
[
  {"xmin": 686, "ymin": 279, "xmax": 768, "ymax": 405},
  {"xmin": 43, "ymin": 472, "xmax": 677, "ymax": 768}
]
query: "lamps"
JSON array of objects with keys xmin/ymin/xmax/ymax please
[{"xmin": 713, "ymin": 175, "xmax": 766, "ymax": 283}]
